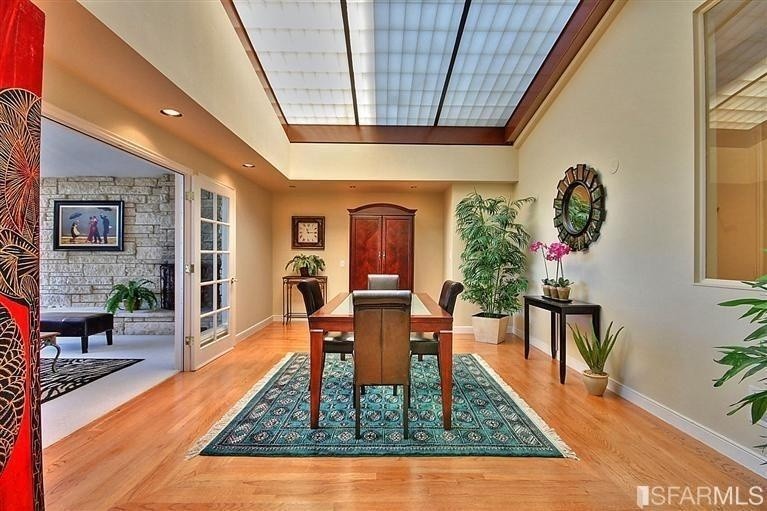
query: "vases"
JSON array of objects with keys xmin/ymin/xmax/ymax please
[
  {"xmin": 549, "ymin": 286, "xmax": 558, "ymax": 298},
  {"xmin": 542, "ymin": 284, "xmax": 550, "ymax": 296},
  {"xmin": 556, "ymin": 287, "xmax": 571, "ymax": 299}
]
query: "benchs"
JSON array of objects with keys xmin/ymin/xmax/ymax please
[{"xmin": 39, "ymin": 310, "xmax": 113, "ymax": 352}]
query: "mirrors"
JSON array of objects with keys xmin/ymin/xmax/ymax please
[{"xmin": 550, "ymin": 161, "xmax": 606, "ymax": 253}]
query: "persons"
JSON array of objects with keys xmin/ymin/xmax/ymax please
[
  {"xmin": 84, "ymin": 215, "xmax": 110, "ymax": 245},
  {"xmin": 70, "ymin": 220, "xmax": 81, "ymax": 241}
]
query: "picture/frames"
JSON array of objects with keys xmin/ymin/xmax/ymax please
[{"xmin": 52, "ymin": 198, "xmax": 125, "ymax": 251}]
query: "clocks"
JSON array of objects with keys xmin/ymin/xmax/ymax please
[{"xmin": 291, "ymin": 214, "xmax": 326, "ymax": 250}]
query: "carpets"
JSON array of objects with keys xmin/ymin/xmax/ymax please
[
  {"xmin": 184, "ymin": 350, "xmax": 580, "ymax": 461},
  {"xmin": 40, "ymin": 356, "xmax": 145, "ymax": 406}
]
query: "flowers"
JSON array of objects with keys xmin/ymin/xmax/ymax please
[{"xmin": 528, "ymin": 240, "xmax": 575, "ymax": 286}]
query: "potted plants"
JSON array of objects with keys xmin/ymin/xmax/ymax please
[
  {"xmin": 285, "ymin": 252, "xmax": 326, "ymax": 276},
  {"xmin": 566, "ymin": 319, "xmax": 625, "ymax": 396},
  {"xmin": 454, "ymin": 180, "xmax": 537, "ymax": 344},
  {"xmin": 103, "ymin": 278, "xmax": 158, "ymax": 315}
]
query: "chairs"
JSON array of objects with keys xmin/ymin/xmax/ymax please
[
  {"xmin": 391, "ymin": 279, "xmax": 465, "ymax": 396},
  {"xmin": 295, "ymin": 277, "xmax": 366, "ymax": 396},
  {"xmin": 351, "ymin": 288, "xmax": 412, "ymax": 439},
  {"xmin": 367, "ymin": 272, "xmax": 400, "ymax": 289}
]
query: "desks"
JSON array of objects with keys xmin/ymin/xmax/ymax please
[
  {"xmin": 282, "ymin": 274, "xmax": 328, "ymax": 325},
  {"xmin": 39, "ymin": 330, "xmax": 62, "ymax": 374},
  {"xmin": 521, "ymin": 294, "xmax": 601, "ymax": 383}
]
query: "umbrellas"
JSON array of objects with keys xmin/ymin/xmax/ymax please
[
  {"xmin": 97, "ymin": 208, "xmax": 113, "ymax": 212},
  {"xmin": 69, "ymin": 212, "xmax": 82, "ymax": 220}
]
